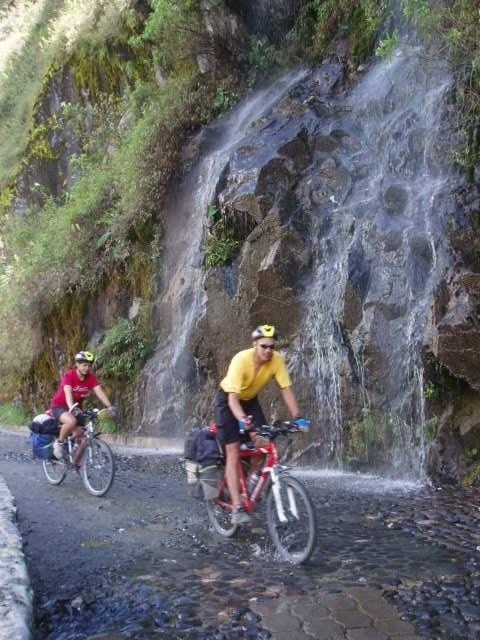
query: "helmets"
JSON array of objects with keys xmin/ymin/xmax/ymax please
[
  {"xmin": 75, "ymin": 350, "xmax": 94, "ymax": 363},
  {"xmin": 251, "ymin": 325, "xmax": 279, "ymax": 340}
]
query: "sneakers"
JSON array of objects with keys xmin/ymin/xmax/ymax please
[
  {"xmin": 76, "ymin": 469, "xmax": 90, "ymax": 481},
  {"xmin": 53, "ymin": 439, "xmax": 63, "ymax": 459},
  {"xmin": 250, "ymin": 470, "xmax": 270, "ymax": 491},
  {"xmin": 230, "ymin": 506, "xmax": 250, "ymax": 524}
]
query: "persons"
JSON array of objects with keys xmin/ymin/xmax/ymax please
[
  {"xmin": 48, "ymin": 349, "xmax": 119, "ymax": 470},
  {"xmin": 213, "ymin": 325, "xmax": 312, "ymax": 527}
]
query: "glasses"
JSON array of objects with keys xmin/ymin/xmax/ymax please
[{"xmin": 260, "ymin": 344, "xmax": 274, "ymax": 349}]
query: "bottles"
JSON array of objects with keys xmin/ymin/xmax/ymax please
[{"xmin": 247, "ymin": 470, "xmax": 262, "ymax": 497}]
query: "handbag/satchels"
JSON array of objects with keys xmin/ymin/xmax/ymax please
[
  {"xmin": 29, "ymin": 414, "xmax": 58, "ymax": 459},
  {"xmin": 185, "ymin": 427, "xmax": 225, "ymax": 500}
]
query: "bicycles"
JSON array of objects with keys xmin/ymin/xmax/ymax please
[
  {"xmin": 43, "ymin": 401, "xmax": 118, "ymax": 497},
  {"xmin": 198, "ymin": 420, "xmax": 320, "ymax": 566}
]
